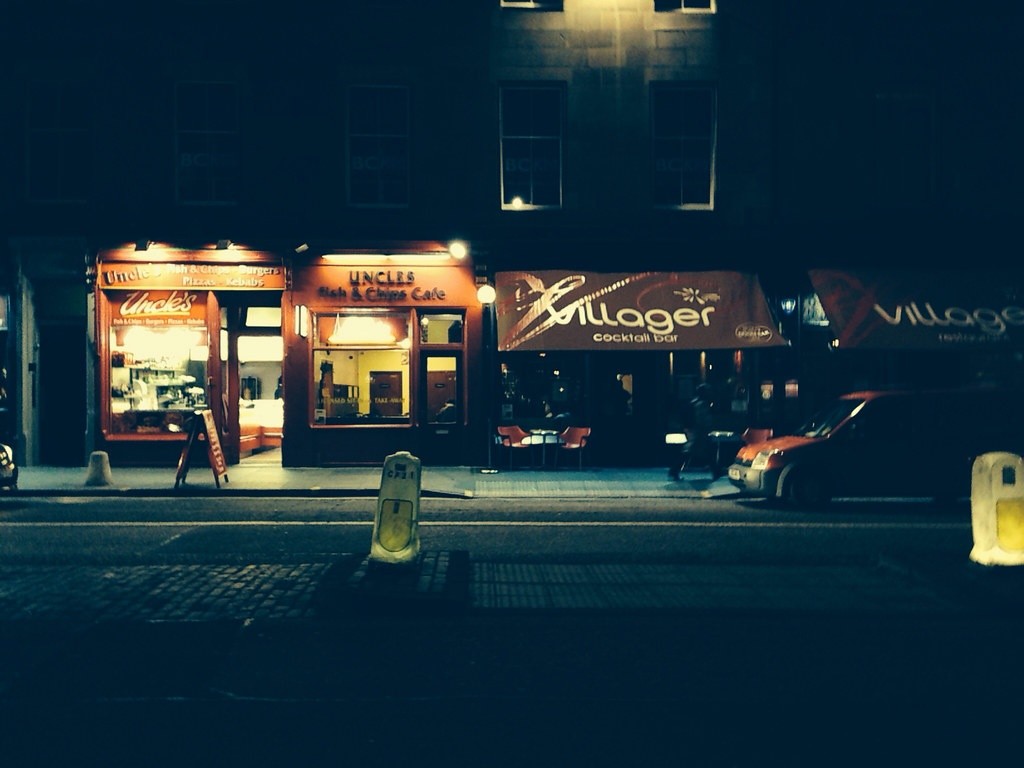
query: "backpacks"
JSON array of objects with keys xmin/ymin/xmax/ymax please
[{"xmin": 679, "ymin": 396, "xmax": 704, "ymax": 429}]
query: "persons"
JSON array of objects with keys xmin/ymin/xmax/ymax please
[{"xmin": 663, "ymin": 381, "xmax": 724, "ymax": 481}]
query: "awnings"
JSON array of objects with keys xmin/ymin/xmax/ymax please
[
  {"xmin": 806, "ymin": 265, "xmax": 1023, "ymax": 351},
  {"xmin": 494, "ymin": 267, "xmax": 792, "ymax": 354}
]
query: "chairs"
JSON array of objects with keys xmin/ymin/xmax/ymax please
[
  {"xmin": 497, "ymin": 425, "xmax": 535, "ymax": 472},
  {"xmin": 554, "ymin": 425, "xmax": 592, "ymax": 472}
]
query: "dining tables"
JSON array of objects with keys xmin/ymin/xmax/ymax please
[{"xmin": 529, "ymin": 429, "xmax": 560, "ymax": 470}]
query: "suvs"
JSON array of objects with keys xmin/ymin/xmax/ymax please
[{"xmin": 726, "ymin": 386, "xmax": 1023, "ymax": 514}]
